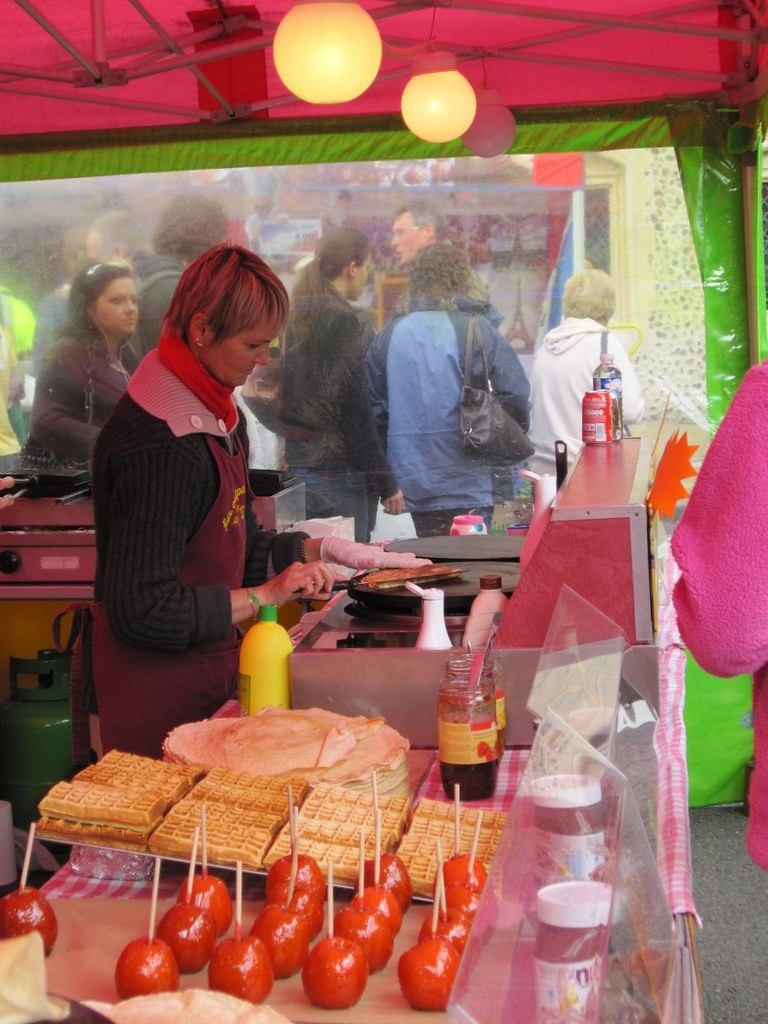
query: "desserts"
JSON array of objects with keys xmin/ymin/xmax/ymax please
[
  {"xmin": 119, "ymin": 851, "xmax": 489, "ymax": 1013},
  {"xmin": 0, "ymin": 886, "xmax": 58, "ymax": 955}
]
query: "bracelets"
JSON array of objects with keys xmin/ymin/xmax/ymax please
[
  {"xmin": 301, "ymin": 539, "xmax": 308, "ymax": 561},
  {"xmin": 245, "ymin": 587, "xmax": 258, "ymax": 623}
]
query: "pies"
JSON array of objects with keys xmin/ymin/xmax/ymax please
[
  {"xmin": 160, "ymin": 706, "xmax": 413, "ymax": 798},
  {"xmin": 358, "ymin": 565, "xmax": 461, "ymax": 590}
]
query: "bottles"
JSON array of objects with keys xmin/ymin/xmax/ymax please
[
  {"xmin": 450, "ymin": 515, "xmax": 486, "ymax": 536},
  {"xmin": 440, "ymin": 648, "xmax": 506, "ymax": 800},
  {"xmin": 506, "ymin": 518, "xmax": 530, "ymax": 535},
  {"xmin": 593, "ymin": 354, "xmax": 623, "ymax": 442},
  {"xmin": 519, "ymin": 469, "xmax": 558, "ymax": 575},
  {"xmin": 532, "ymin": 773, "xmax": 606, "ymax": 893},
  {"xmin": 531, "ymin": 880, "xmax": 603, "ymax": 1024},
  {"xmin": 404, "ymin": 581, "xmax": 453, "ymax": 648},
  {"xmin": 463, "ymin": 575, "xmax": 510, "ymax": 648},
  {"xmin": 238, "ymin": 590, "xmax": 293, "ymax": 717}
]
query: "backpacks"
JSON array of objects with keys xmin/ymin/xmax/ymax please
[{"xmin": 239, "ymin": 319, "xmax": 326, "ymax": 445}]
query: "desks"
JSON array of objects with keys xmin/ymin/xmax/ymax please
[{"xmin": 36, "ymin": 748, "xmax": 623, "ymax": 1024}]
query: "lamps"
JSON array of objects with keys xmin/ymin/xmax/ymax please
[
  {"xmin": 272, "ymin": 0, "xmax": 383, "ymax": 104},
  {"xmin": 400, "ymin": 0, "xmax": 477, "ymax": 145},
  {"xmin": 462, "ymin": 48, "xmax": 516, "ymax": 158}
]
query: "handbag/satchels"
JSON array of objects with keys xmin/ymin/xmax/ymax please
[
  {"xmin": 5, "ymin": 401, "xmax": 27, "ymax": 447},
  {"xmin": 461, "ymin": 314, "xmax": 535, "ymax": 466},
  {"xmin": 20, "ymin": 340, "xmax": 94, "ymax": 469}
]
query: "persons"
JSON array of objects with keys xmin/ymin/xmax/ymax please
[
  {"xmin": 115, "ymin": 197, "xmax": 230, "ymax": 373},
  {"xmin": 0, "ymin": 196, "xmax": 302, "ymax": 587},
  {"xmin": 362, "ymin": 243, "xmax": 534, "ymax": 537},
  {"xmin": 392, "ymin": 200, "xmax": 490, "ymax": 311},
  {"xmin": 276, "ymin": 228, "xmax": 403, "ymax": 542},
  {"xmin": 671, "ymin": 357, "xmax": 768, "ymax": 869},
  {"xmin": 87, "ymin": 210, "xmax": 147, "ymax": 261},
  {"xmin": 529, "ymin": 269, "xmax": 645, "ymax": 476},
  {"xmin": 91, "ymin": 247, "xmax": 434, "ymax": 761}
]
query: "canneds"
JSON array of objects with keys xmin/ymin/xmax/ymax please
[
  {"xmin": 533, "ymin": 879, "xmax": 624, "ymax": 1024},
  {"xmin": 581, "ymin": 391, "xmax": 612, "ymax": 445},
  {"xmin": 529, "ymin": 774, "xmax": 606, "ymax": 888},
  {"xmin": 437, "ymin": 646, "xmax": 506, "ymax": 801}
]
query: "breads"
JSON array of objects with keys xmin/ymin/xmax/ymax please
[{"xmin": 35, "ymin": 750, "xmax": 508, "ymax": 900}]
